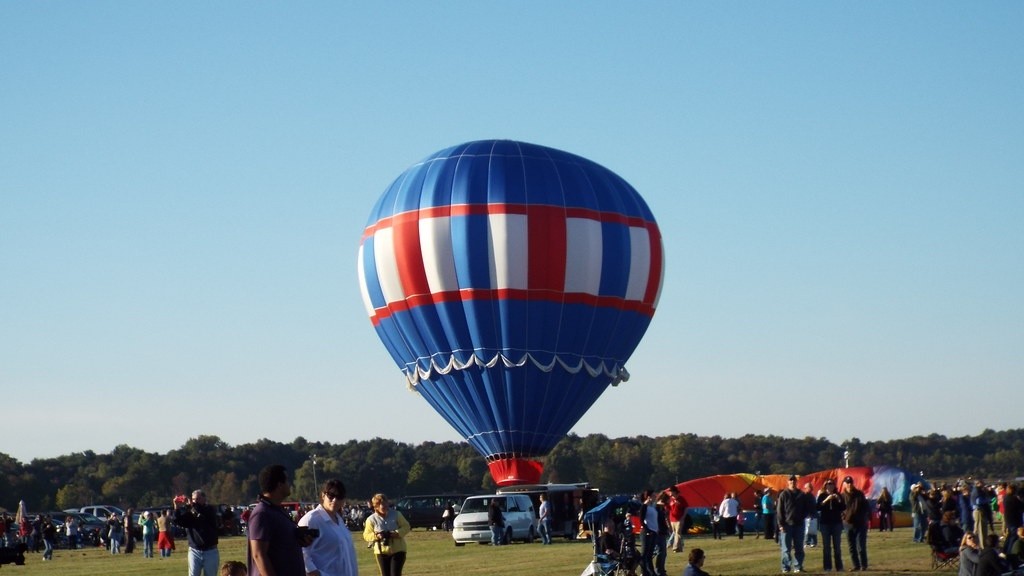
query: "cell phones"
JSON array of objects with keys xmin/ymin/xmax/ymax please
[{"xmin": 175, "ymin": 496, "xmax": 184, "ymax": 503}]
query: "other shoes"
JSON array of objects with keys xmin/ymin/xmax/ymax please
[
  {"xmin": 848, "ymin": 566, "xmax": 861, "ymax": 572},
  {"xmin": 143, "ymin": 556, "xmax": 148, "ymax": 558},
  {"xmin": 837, "ymin": 569, "xmax": 846, "ymax": 572},
  {"xmin": 672, "ymin": 549, "xmax": 683, "ymax": 553},
  {"xmin": 810, "ymin": 544, "xmax": 816, "ymax": 548},
  {"xmin": 860, "ymin": 567, "xmax": 867, "ymax": 571},
  {"xmin": 794, "ymin": 569, "xmax": 808, "ymax": 575},
  {"xmin": 150, "ymin": 556, "xmax": 153, "ymax": 559},
  {"xmin": 803, "ymin": 545, "xmax": 810, "ymax": 548},
  {"xmin": 42, "ymin": 557, "xmax": 46, "ymax": 561},
  {"xmin": 825, "ymin": 569, "xmax": 832, "ymax": 573},
  {"xmin": 783, "ymin": 570, "xmax": 789, "ymax": 575}
]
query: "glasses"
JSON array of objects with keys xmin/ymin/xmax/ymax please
[
  {"xmin": 702, "ymin": 556, "xmax": 706, "ymax": 559},
  {"xmin": 950, "ymin": 518, "xmax": 955, "ymax": 520},
  {"xmin": 968, "ymin": 536, "xmax": 975, "ymax": 539},
  {"xmin": 323, "ymin": 490, "xmax": 345, "ymax": 501}
]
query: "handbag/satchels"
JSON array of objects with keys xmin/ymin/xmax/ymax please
[{"xmin": 926, "ymin": 521, "xmax": 949, "ymax": 548}]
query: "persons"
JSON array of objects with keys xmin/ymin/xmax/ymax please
[
  {"xmin": 878, "ymin": 487, "xmax": 893, "ymax": 532},
  {"xmin": 221, "ymin": 561, "xmax": 247, "ymax": 576},
  {"xmin": 816, "ymin": 479, "xmax": 846, "ymax": 572},
  {"xmin": 363, "ymin": 494, "xmax": 410, "ymax": 576},
  {"xmin": 909, "ymin": 477, "xmax": 1024, "ymax": 576},
  {"xmin": 0, "ymin": 508, "xmax": 176, "ymax": 566},
  {"xmin": 171, "ymin": 490, "xmax": 220, "ymax": 576},
  {"xmin": 537, "ymin": 494, "xmax": 552, "ymax": 544},
  {"xmin": 488, "ymin": 498, "xmax": 506, "ymax": 546},
  {"xmin": 452, "ymin": 501, "xmax": 461, "ymax": 515},
  {"xmin": 710, "ymin": 483, "xmax": 822, "ymax": 548},
  {"xmin": 297, "ymin": 481, "xmax": 359, "ymax": 576},
  {"xmin": 578, "ymin": 485, "xmax": 689, "ymax": 576},
  {"xmin": 444, "ymin": 502, "xmax": 454, "ymax": 532},
  {"xmin": 777, "ymin": 475, "xmax": 809, "ymax": 573},
  {"xmin": 684, "ymin": 548, "xmax": 711, "ymax": 576},
  {"xmin": 221, "ymin": 501, "xmax": 374, "ymax": 536},
  {"xmin": 247, "ymin": 464, "xmax": 319, "ymax": 576},
  {"xmin": 840, "ymin": 476, "xmax": 870, "ymax": 572}
]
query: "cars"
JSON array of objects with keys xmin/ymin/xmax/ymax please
[{"xmin": 0, "ymin": 503, "xmax": 256, "ymax": 549}]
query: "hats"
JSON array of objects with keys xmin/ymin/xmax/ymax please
[
  {"xmin": 668, "ymin": 486, "xmax": 680, "ymax": 492},
  {"xmin": 843, "ymin": 476, "xmax": 853, "ymax": 483},
  {"xmin": 788, "ymin": 473, "xmax": 796, "ymax": 481},
  {"xmin": 910, "ymin": 484, "xmax": 919, "ymax": 490}
]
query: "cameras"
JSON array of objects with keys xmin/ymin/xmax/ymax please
[
  {"xmin": 297, "ymin": 522, "xmax": 320, "ymax": 547},
  {"xmin": 379, "ymin": 531, "xmax": 391, "ymax": 546}
]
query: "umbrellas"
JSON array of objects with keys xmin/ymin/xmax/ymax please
[{"xmin": 15, "ymin": 500, "xmax": 27, "ymax": 524}]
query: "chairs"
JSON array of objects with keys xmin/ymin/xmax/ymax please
[
  {"xmin": 593, "ymin": 536, "xmax": 641, "ymax": 576},
  {"xmin": 927, "ymin": 521, "xmax": 960, "ymax": 572}
]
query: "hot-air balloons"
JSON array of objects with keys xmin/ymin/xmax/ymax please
[{"xmin": 358, "ymin": 138, "xmax": 665, "ymax": 491}]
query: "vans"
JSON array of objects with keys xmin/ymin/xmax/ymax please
[
  {"xmin": 388, "ymin": 496, "xmax": 454, "ymax": 530},
  {"xmin": 452, "ymin": 494, "xmax": 538, "ymax": 547}
]
